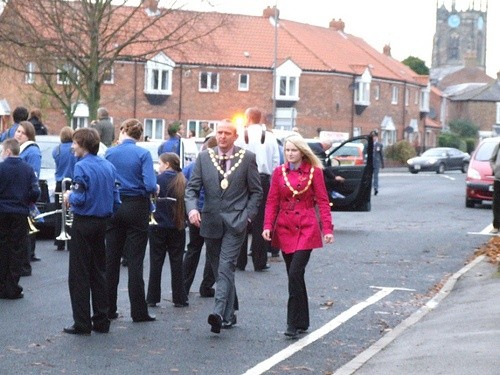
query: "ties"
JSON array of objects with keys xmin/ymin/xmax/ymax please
[{"xmin": 218, "ymin": 154, "xmax": 226, "ymax": 182}]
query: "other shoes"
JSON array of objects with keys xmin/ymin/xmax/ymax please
[
  {"xmin": 173, "ymin": 302, "xmax": 189, "ymax": 307},
  {"xmin": 374, "ymin": 188, "xmax": 378, "ymax": 196},
  {"xmin": 284, "ymin": 327, "xmax": 310, "ymax": 337},
  {"xmin": 33, "ymin": 255, "xmax": 40, "ymax": 261},
  {"xmin": 146, "ymin": 301, "xmax": 157, "ymax": 307},
  {"xmin": 110, "ymin": 312, "xmax": 118, "ymax": 318},
  {"xmin": 208, "ymin": 313, "xmax": 222, "ymax": 334},
  {"xmin": 4, "ymin": 286, "xmax": 23, "ymax": 299},
  {"xmin": 254, "ymin": 263, "xmax": 271, "ymax": 271},
  {"xmin": 222, "ymin": 314, "xmax": 237, "ymax": 326}
]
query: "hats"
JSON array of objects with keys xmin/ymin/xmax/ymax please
[{"xmin": 369, "ymin": 131, "xmax": 379, "ymax": 137}]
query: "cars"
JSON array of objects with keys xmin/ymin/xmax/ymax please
[
  {"xmin": 274, "ymin": 132, "xmax": 378, "ymax": 177},
  {"xmin": 30, "ymin": 135, "xmax": 107, "ymax": 238},
  {"xmin": 132, "ymin": 137, "xmax": 196, "ymax": 173},
  {"xmin": 406, "ymin": 147, "xmax": 472, "ymax": 175},
  {"xmin": 322, "ymin": 142, "xmax": 364, "ymax": 169},
  {"xmin": 465, "ymin": 136, "xmax": 500, "ymax": 208}
]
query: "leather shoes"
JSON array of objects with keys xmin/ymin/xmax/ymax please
[
  {"xmin": 64, "ymin": 325, "xmax": 91, "ymax": 334},
  {"xmin": 133, "ymin": 312, "xmax": 155, "ymax": 322}
]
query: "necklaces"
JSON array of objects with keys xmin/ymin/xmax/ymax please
[
  {"xmin": 281, "ymin": 164, "xmax": 315, "ymax": 197},
  {"xmin": 206, "ymin": 147, "xmax": 247, "ymax": 190}
]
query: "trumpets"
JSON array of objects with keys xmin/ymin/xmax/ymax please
[
  {"xmin": 150, "ymin": 181, "xmax": 159, "ymax": 229},
  {"xmin": 55, "ymin": 177, "xmax": 74, "ymax": 241},
  {"xmin": 26, "ymin": 212, "xmax": 40, "ymax": 237}
]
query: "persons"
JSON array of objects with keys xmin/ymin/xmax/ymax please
[
  {"xmin": 185, "ymin": 121, "xmax": 263, "ymax": 334},
  {"xmin": 14, "ymin": 121, "xmax": 42, "ymax": 262},
  {"xmin": 261, "ymin": 133, "xmax": 334, "ymax": 336},
  {"xmin": 147, "ymin": 153, "xmax": 190, "ymax": 307},
  {"xmin": 489, "ymin": 142, "xmax": 500, "ymax": 230},
  {"xmin": 233, "ymin": 107, "xmax": 284, "ymax": 272},
  {"xmin": 308, "ymin": 139, "xmax": 345, "ymax": 183},
  {"xmin": 0, "ymin": 107, "xmax": 29, "ymax": 142},
  {"xmin": 90, "ymin": 108, "xmax": 115, "ymax": 147},
  {"xmin": 182, "ymin": 137, "xmax": 216, "ymax": 298},
  {"xmin": 52, "ymin": 126, "xmax": 78, "ymax": 251},
  {"xmin": 62, "ymin": 127, "xmax": 121, "ymax": 335},
  {"xmin": 103, "ymin": 119, "xmax": 158, "ymax": 322},
  {"xmin": 363, "ymin": 131, "xmax": 384, "ymax": 196},
  {"xmin": 0, "ymin": 138, "xmax": 40, "ymax": 300},
  {"xmin": 159, "ymin": 120, "xmax": 215, "ymax": 165},
  {"xmin": 29, "ymin": 109, "xmax": 47, "ymax": 135}
]
